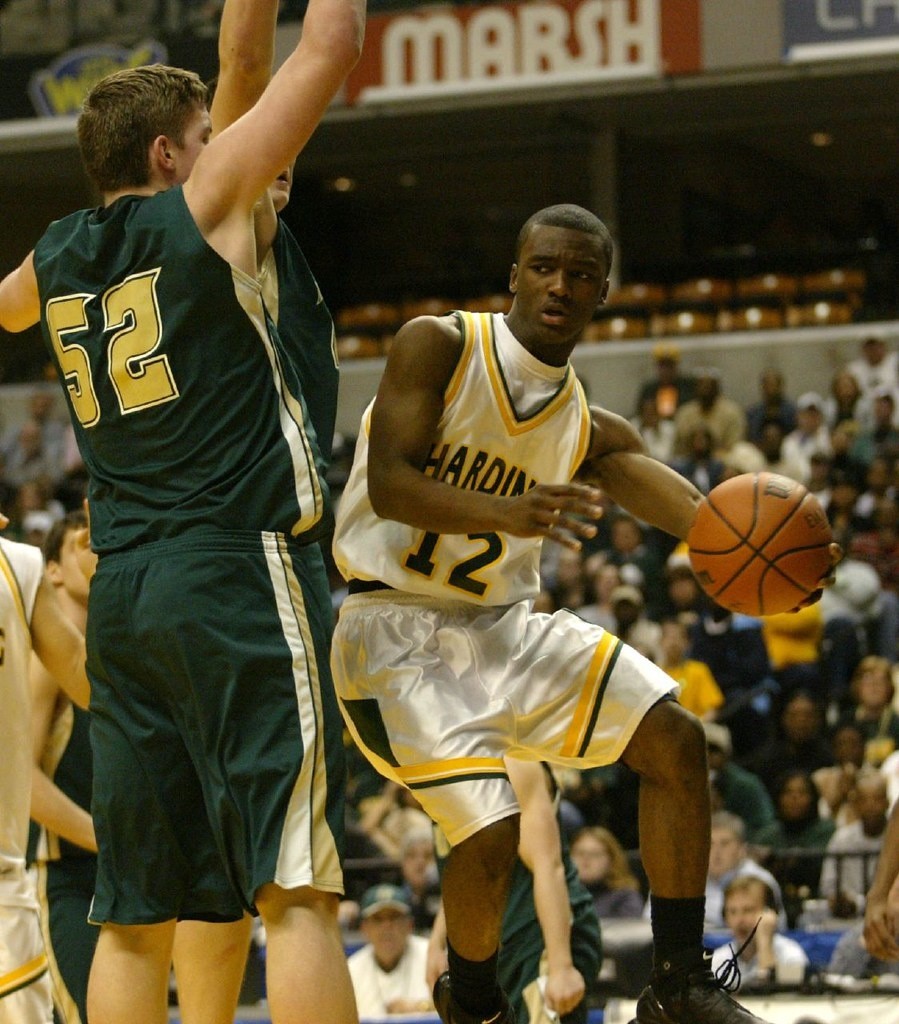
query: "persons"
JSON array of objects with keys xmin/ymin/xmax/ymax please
[
  {"xmin": 315, "ymin": 326, "xmax": 899, "ymax": 1024},
  {"xmin": 1, "ymin": 0, "xmax": 369, "ymax": 1024},
  {"xmin": 328, "ymin": 202, "xmax": 842, "ymax": 1023}
]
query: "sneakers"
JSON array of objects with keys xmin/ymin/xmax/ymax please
[
  {"xmin": 636, "ymin": 916, "xmax": 775, "ymax": 1024},
  {"xmin": 432, "ymin": 971, "xmax": 517, "ymax": 1024}
]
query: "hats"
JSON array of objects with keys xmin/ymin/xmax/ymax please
[
  {"xmin": 608, "ymin": 585, "xmax": 642, "ymax": 607},
  {"xmin": 359, "ymin": 884, "xmax": 413, "ymax": 917},
  {"xmin": 798, "ymin": 392, "xmax": 823, "ymax": 414},
  {"xmin": 704, "ymin": 722, "xmax": 733, "ymax": 756}
]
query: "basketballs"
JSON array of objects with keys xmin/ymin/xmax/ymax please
[{"xmin": 687, "ymin": 470, "xmax": 834, "ymax": 617}]
